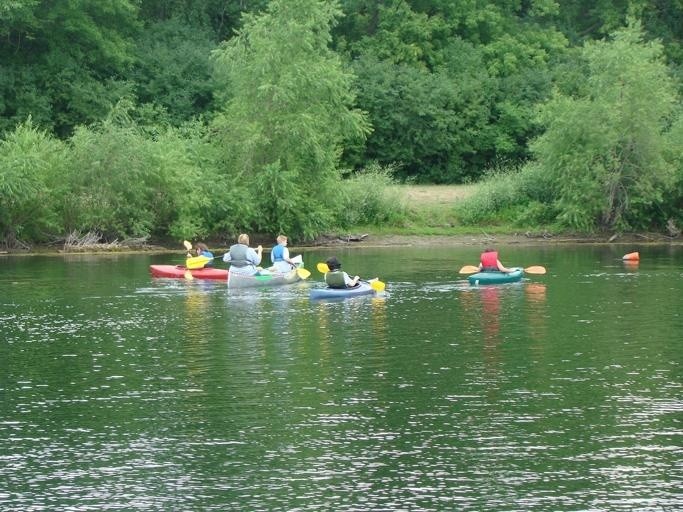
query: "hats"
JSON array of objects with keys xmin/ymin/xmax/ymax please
[{"xmin": 326, "ymin": 257, "xmax": 341, "ymax": 270}]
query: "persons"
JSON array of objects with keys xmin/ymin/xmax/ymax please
[
  {"xmin": 271, "ymin": 235, "xmax": 296, "ymax": 273},
  {"xmin": 186, "ymin": 242, "xmax": 213, "ymax": 266},
  {"xmin": 222, "ymin": 233, "xmax": 271, "ymax": 276},
  {"xmin": 477, "ymin": 246, "xmax": 516, "ymax": 272},
  {"xmin": 323, "ymin": 257, "xmax": 360, "ymax": 287}
]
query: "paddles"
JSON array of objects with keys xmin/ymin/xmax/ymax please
[
  {"xmin": 459, "ymin": 265, "xmax": 547, "ymax": 274},
  {"xmin": 184, "ymin": 241, "xmax": 194, "ymax": 280},
  {"xmin": 317, "ymin": 262, "xmax": 386, "ymax": 291},
  {"xmin": 288, "ymin": 261, "xmax": 311, "ymax": 280},
  {"xmin": 186, "ymin": 246, "xmax": 262, "ymax": 270}
]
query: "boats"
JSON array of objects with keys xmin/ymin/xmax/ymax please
[
  {"xmin": 307, "ymin": 277, "xmax": 381, "ymax": 302},
  {"xmin": 227, "ymin": 254, "xmax": 305, "ymax": 290},
  {"xmin": 468, "ymin": 265, "xmax": 526, "ymax": 286},
  {"xmin": 147, "ymin": 263, "xmax": 228, "ymax": 281}
]
